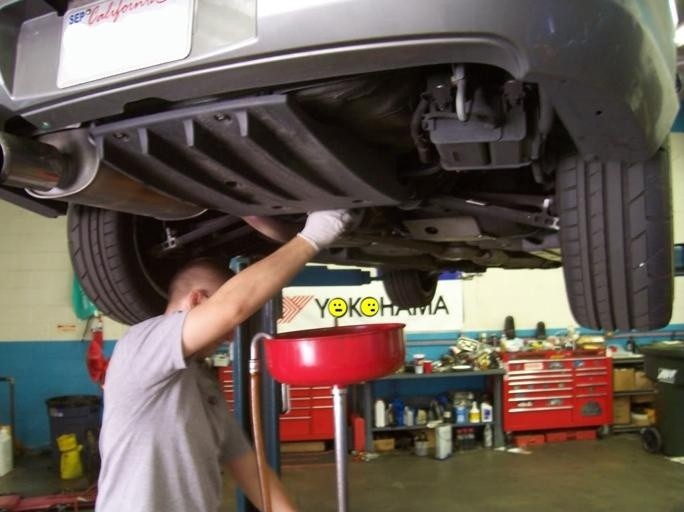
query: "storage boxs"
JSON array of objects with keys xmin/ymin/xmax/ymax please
[
  {"xmin": 636, "ymin": 369, "xmax": 653, "ymax": 391},
  {"xmin": 613, "ymin": 366, "xmax": 636, "ymax": 391},
  {"xmin": 614, "ymin": 397, "xmax": 632, "ymax": 425}
]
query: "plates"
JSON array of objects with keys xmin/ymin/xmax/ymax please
[
  {"xmin": 612, "ymin": 354, "xmax": 646, "ymax": 358},
  {"xmin": 662, "ymin": 340, "xmax": 681, "ymax": 344},
  {"xmin": 452, "ymin": 365, "xmax": 471, "ymax": 370}
]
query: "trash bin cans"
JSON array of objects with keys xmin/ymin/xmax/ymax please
[{"xmin": 636, "ymin": 339, "xmax": 684, "ymax": 457}]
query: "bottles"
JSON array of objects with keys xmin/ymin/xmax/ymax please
[
  {"xmin": 626, "ymin": 335, "xmax": 634, "ymax": 352},
  {"xmin": 456, "ymin": 428, "xmax": 474, "ymax": 449},
  {"xmin": 373, "ymin": 392, "xmax": 493, "ymax": 427},
  {"xmin": 483, "ymin": 423, "xmax": 493, "ymax": 447},
  {"xmin": 491, "ymin": 333, "xmax": 496, "ymax": 346},
  {"xmin": 424, "ymin": 360, "xmax": 431, "ymax": 374},
  {"xmin": 500, "ymin": 334, "xmax": 508, "ymax": 340},
  {"xmin": 480, "ymin": 333, "xmax": 486, "ymax": 344},
  {"xmin": 413, "ymin": 354, "xmax": 425, "ymax": 374}
]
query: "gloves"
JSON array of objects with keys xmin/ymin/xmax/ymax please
[{"xmin": 297, "ymin": 208, "xmax": 352, "ymax": 251}]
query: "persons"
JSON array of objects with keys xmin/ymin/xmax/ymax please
[{"xmin": 92, "ymin": 210, "xmax": 352, "ymax": 512}]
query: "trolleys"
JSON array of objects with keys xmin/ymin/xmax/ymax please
[{"xmin": 502, "ymin": 351, "xmax": 613, "ymax": 443}]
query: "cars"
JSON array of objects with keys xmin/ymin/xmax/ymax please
[{"xmin": 1, "ymin": 0, "xmax": 683, "ymax": 333}]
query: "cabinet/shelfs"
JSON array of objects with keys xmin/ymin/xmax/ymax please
[
  {"xmin": 362, "ymin": 370, "xmax": 503, "ymax": 454},
  {"xmin": 613, "ymin": 357, "xmax": 658, "ymax": 434}
]
